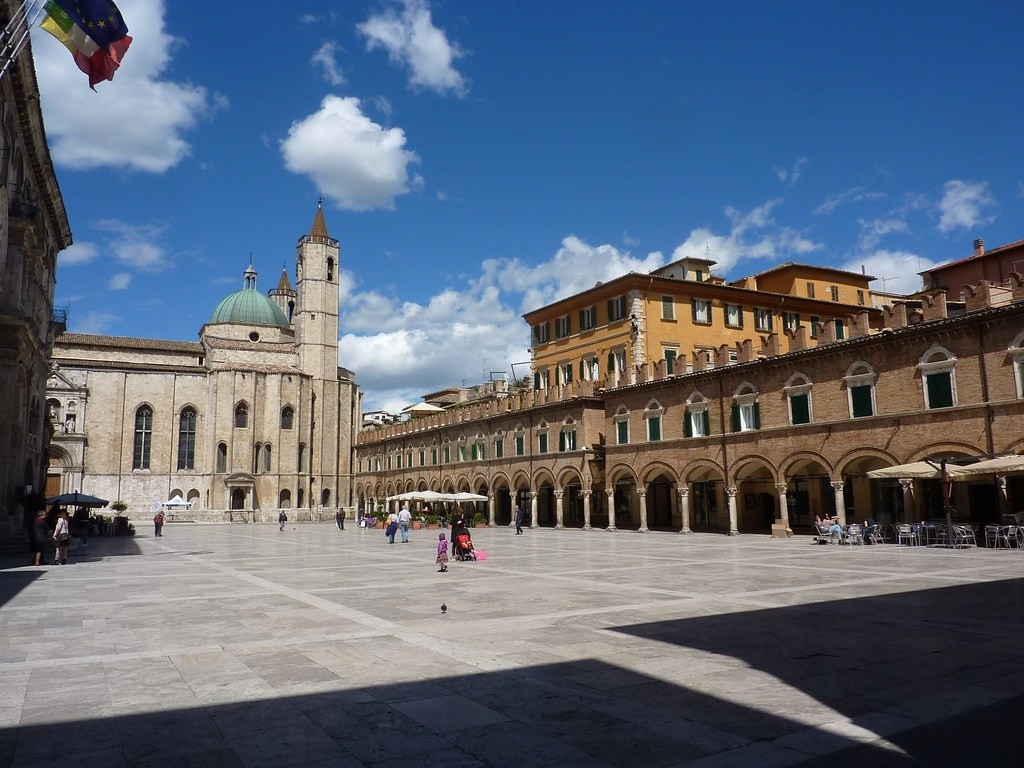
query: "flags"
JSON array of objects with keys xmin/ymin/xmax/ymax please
[{"xmin": 40, "ymin": 0, "xmax": 134, "ymax": 93}]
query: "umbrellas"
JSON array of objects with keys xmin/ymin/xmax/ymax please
[
  {"xmin": 45, "ymin": 492, "xmax": 109, "ymax": 512},
  {"xmin": 949, "ymin": 454, "xmax": 1024, "ymax": 524},
  {"xmin": 867, "ymin": 460, "xmax": 962, "ymax": 520},
  {"xmin": 387, "ymin": 490, "xmax": 489, "ymax": 527}
]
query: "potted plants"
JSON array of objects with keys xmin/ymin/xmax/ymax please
[
  {"xmin": 426, "ymin": 515, "xmax": 440, "ymax": 529},
  {"xmin": 473, "ymin": 513, "xmax": 487, "ymax": 527},
  {"xmin": 446, "ymin": 515, "xmax": 452, "ymax": 529},
  {"xmin": 411, "ymin": 514, "xmax": 422, "ymax": 531},
  {"xmin": 372, "ymin": 497, "xmax": 389, "ymax": 529},
  {"xmin": 102, "ymin": 501, "xmax": 136, "ymax": 536}
]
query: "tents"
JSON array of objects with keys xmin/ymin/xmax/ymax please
[
  {"xmin": 399, "ymin": 402, "xmax": 446, "ymax": 423},
  {"xmin": 163, "ymin": 494, "xmax": 192, "ymax": 510}
]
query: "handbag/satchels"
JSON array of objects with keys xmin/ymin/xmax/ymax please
[{"xmin": 58, "ymin": 533, "xmax": 70, "ymax": 545}]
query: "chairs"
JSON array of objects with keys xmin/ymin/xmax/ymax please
[{"xmin": 815, "ymin": 518, "xmax": 1024, "ymax": 549}]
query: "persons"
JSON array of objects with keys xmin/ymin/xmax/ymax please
[
  {"xmin": 398, "ymin": 505, "xmax": 412, "ymax": 543},
  {"xmin": 30, "ymin": 500, "xmax": 105, "ymax": 566},
  {"xmin": 449, "ymin": 507, "xmax": 465, "ymax": 558},
  {"xmin": 436, "ymin": 533, "xmax": 448, "ymax": 572},
  {"xmin": 279, "ymin": 510, "xmax": 287, "ymax": 531},
  {"xmin": 861, "ymin": 519, "xmax": 876, "ymax": 545},
  {"xmin": 385, "ymin": 509, "xmax": 400, "ymax": 544},
  {"xmin": 514, "ymin": 506, "xmax": 523, "ymax": 535},
  {"xmin": 815, "ymin": 513, "xmax": 849, "ymax": 544},
  {"xmin": 67, "ymin": 417, "xmax": 75, "ymax": 433},
  {"xmin": 154, "ymin": 510, "xmax": 164, "ymax": 537},
  {"xmin": 336, "ymin": 507, "xmax": 345, "ymax": 531}
]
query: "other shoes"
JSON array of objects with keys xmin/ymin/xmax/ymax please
[
  {"xmin": 444, "ymin": 566, "xmax": 447, "ymax": 570},
  {"xmin": 438, "ymin": 569, "xmax": 444, "ymax": 572},
  {"xmin": 515, "ymin": 533, "xmax": 519, "ymax": 535},
  {"xmin": 406, "ymin": 540, "xmax": 408, "ymax": 542},
  {"xmin": 402, "ymin": 541, "xmax": 406, "ymax": 543},
  {"xmin": 521, "ymin": 530, "xmax": 523, "ymax": 534}
]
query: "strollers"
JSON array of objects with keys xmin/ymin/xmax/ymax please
[{"xmin": 454, "ymin": 522, "xmax": 477, "ymax": 562}]
